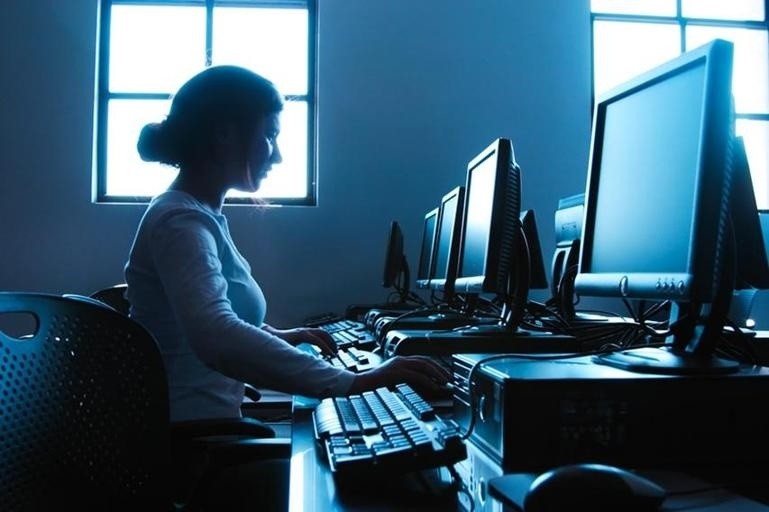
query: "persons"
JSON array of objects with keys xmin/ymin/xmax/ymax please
[{"xmin": 122, "ymin": 66, "xmax": 453, "ymax": 438}]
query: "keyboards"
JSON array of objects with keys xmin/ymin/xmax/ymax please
[{"xmin": 288, "ymin": 309, "xmax": 467, "ymax": 482}]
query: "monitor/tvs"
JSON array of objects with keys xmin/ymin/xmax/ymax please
[
  {"xmin": 569, "ymin": 38, "xmax": 742, "ymax": 373},
  {"xmin": 382, "ymin": 137, "xmax": 549, "ymax": 335},
  {"xmin": 540, "ymin": 192, "xmax": 609, "ymax": 322}
]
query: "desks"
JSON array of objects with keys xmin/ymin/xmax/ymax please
[{"xmin": 289, "ymin": 331, "xmax": 768, "ymax": 510}]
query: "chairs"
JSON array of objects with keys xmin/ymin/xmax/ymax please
[{"xmin": 1, "ymin": 283, "xmax": 171, "ymax": 511}]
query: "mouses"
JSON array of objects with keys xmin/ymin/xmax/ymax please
[{"xmin": 522, "ymin": 459, "xmax": 667, "ymax": 512}]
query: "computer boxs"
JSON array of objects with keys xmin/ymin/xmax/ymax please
[
  {"xmin": 345, "ymin": 303, "xmax": 578, "ymax": 360},
  {"xmin": 451, "ymin": 350, "xmax": 769, "ymax": 473}
]
